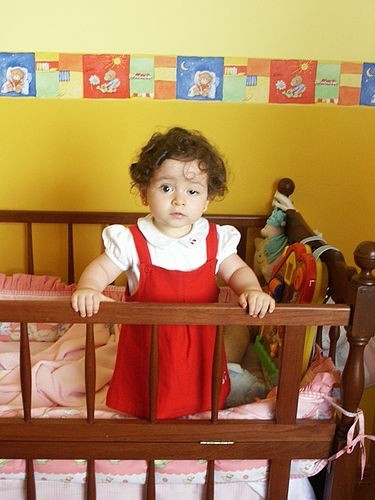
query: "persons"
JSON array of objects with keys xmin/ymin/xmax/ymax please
[{"xmin": 71, "ymin": 125, "xmax": 279, "ymax": 421}]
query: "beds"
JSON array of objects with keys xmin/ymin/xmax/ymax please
[{"xmin": 0, "ymin": 178, "xmax": 375, "ymax": 500}]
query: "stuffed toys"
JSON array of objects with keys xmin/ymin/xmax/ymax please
[{"xmin": 252, "ymin": 210, "xmax": 291, "ymax": 282}]
